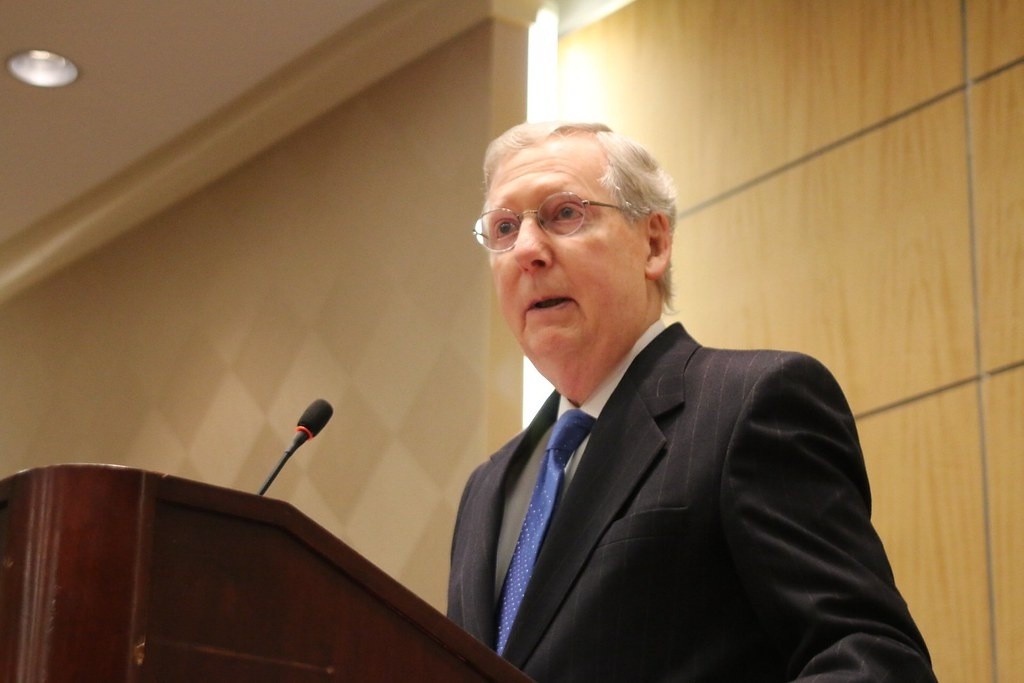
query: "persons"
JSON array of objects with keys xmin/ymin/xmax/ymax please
[{"xmin": 445, "ymin": 119, "xmax": 937, "ymax": 683}]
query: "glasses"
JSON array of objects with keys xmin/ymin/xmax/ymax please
[{"xmin": 471, "ymin": 190, "xmax": 628, "ymax": 253}]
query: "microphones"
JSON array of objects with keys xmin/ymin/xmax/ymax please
[{"xmin": 259, "ymin": 398, "xmax": 332, "ymax": 497}]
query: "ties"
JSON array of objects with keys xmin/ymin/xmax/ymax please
[{"xmin": 497, "ymin": 409, "xmax": 597, "ymax": 657}]
